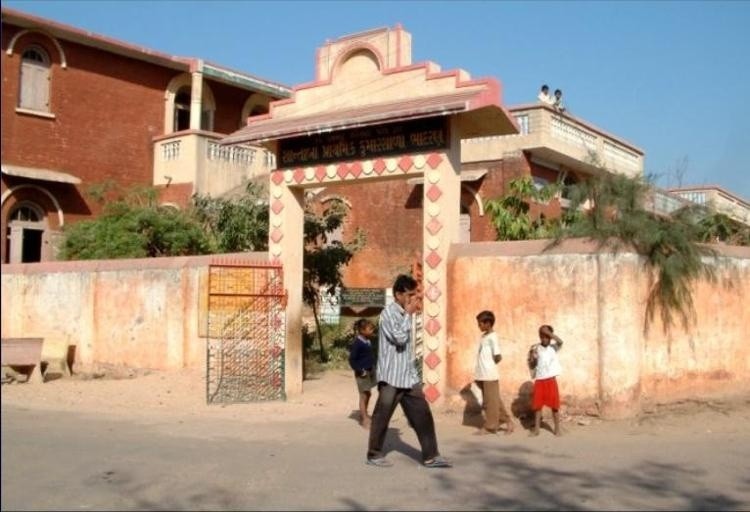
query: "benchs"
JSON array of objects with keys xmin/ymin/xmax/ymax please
[{"xmin": 1, "ymin": 336, "xmax": 72, "ymax": 384}]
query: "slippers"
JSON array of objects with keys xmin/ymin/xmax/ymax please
[
  {"xmin": 366, "ymin": 458, "xmax": 393, "ymax": 467},
  {"xmin": 424, "ymin": 457, "xmax": 454, "ymax": 468}
]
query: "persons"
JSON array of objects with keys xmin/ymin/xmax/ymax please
[
  {"xmin": 526, "ymin": 324, "xmax": 564, "ymax": 438},
  {"xmin": 472, "ymin": 310, "xmax": 517, "ymax": 437},
  {"xmin": 550, "ymin": 89, "xmax": 566, "ymax": 115},
  {"xmin": 363, "ymin": 274, "xmax": 455, "ymax": 468},
  {"xmin": 348, "ymin": 318, "xmax": 377, "ymax": 430},
  {"xmin": 537, "ymin": 85, "xmax": 555, "ymax": 105}
]
